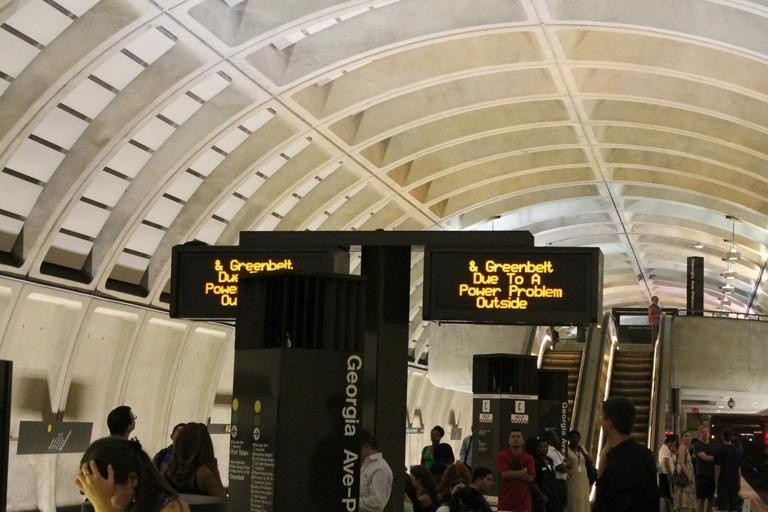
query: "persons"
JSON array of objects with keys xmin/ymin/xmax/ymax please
[
  {"xmin": 151, "ymin": 422, "xmax": 187, "ymax": 475},
  {"xmin": 74, "ymin": 436, "xmax": 189, "ymax": 512},
  {"xmin": 647, "ymin": 295, "xmax": 662, "ymax": 359},
  {"xmin": 80, "ymin": 405, "xmax": 134, "ymax": 512},
  {"xmin": 589, "ymin": 401, "xmax": 616, "ymax": 504},
  {"xmin": 657, "ymin": 424, "xmax": 742, "ymax": 512},
  {"xmin": 594, "ymin": 396, "xmax": 660, "ymax": 512},
  {"xmin": 162, "ymin": 422, "xmax": 228, "ymax": 496},
  {"xmin": 358, "ymin": 430, "xmax": 395, "ymax": 512},
  {"xmin": 404, "ymin": 425, "xmax": 597, "ymax": 512}
]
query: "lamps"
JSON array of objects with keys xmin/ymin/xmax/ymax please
[{"xmin": 719, "ymin": 217, "xmax": 741, "ymax": 320}]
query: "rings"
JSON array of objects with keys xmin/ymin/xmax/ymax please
[{"xmin": 83, "ymin": 472, "xmax": 89, "ymax": 477}]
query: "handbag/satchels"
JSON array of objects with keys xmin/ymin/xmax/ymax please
[
  {"xmin": 672, "ymin": 469, "xmax": 688, "ymax": 487},
  {"xmin": 586, "ymin": 459, "xmax": 598, "ymax": 485},
  {"xmin": 658, "ymin": 473, "xmax": 670, "ymax": 497}
]
